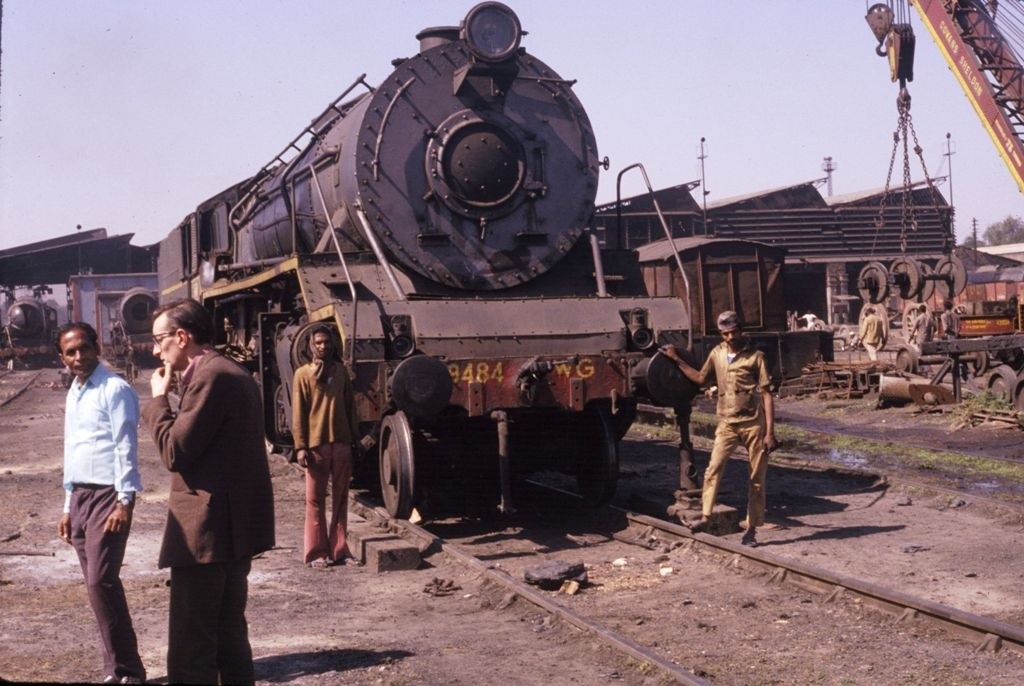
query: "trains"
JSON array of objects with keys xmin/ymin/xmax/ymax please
[{"xmin": 151, "ymin": 0, "xmax": 705, "ymax": 520}]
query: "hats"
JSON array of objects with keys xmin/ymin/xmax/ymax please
[
  {"xmin": 864, "ymin": 307, "xmax": 876, "ymax": 317},
  {"xmin": 717, "ymin": 310, "xmax": 739, "ymax": 333}
]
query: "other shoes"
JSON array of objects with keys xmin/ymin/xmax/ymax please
[
  {"xmin": 690, "ymin": 517, "xmax": 710, "ymax": 532},
  {"xmin": 103, "ymin": 673, "xmax": 144, "ymax": 685},
  {"xmin": 334, "ymin": 556, "xmax": 363, "ymax": 568},
  {"xmin": 309, "ymin": 556, "xmax": 327, "ymax": 569},
  {"xmin": 739, "ymin": 527, "xmax": 755, "ymax": 544}
]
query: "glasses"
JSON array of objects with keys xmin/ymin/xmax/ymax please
[{"xmin": 151, "ymin": 331, "xmax": 190, "ymax": 347}]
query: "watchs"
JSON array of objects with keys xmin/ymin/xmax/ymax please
[{"xmin": 115, "ymin": 496, "xmax": 134, "ymax": 510}]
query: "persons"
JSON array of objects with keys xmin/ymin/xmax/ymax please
[
  {"xmin": 141, "ymin": 306, "xmax": 275, "ymax": 686},
  {"xmin": 908, "ymin": 304, "xmax": 938, "ymax": 373},
  {"xmin": 291, "ymin": 325, "xmax": 362, "ymax": 571},
  {"xmin": 798, "ymin": 309, "xmax": 818, "ymax": 329},
  {"xmin": 657, "ymin": 310, "xmax": 777, "ymax": 548},
  {"xmin": 941, "ymin": 301, "xmax": 958, "ymax": 340},
  {"xmin": 55, "ymin": 322, "xmax": 146, "ymax": 686},
  {"xmin": 858, "ymin": 305, "xmax": 886, "ymax": 361}
]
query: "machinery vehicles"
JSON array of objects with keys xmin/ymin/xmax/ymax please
[{"xmin": 861, "ymin": 0, "xmax": 1024, "ymax": 430}]
